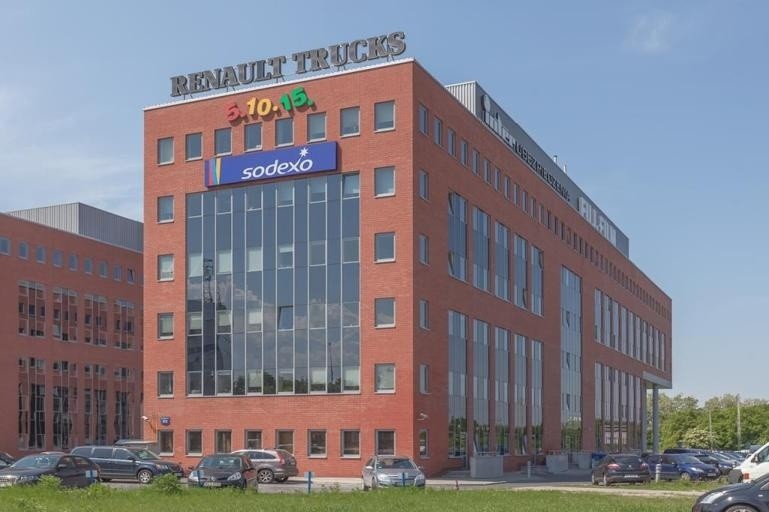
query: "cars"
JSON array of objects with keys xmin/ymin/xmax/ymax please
[
  {"xmin": 591, "ymin": 440, "xmax": 769, "ymax": 512},
  {"xmin": 360, "ymin": 454, "xmax": 426, "ymax": 492},
  {"xmin": 1, "ymin": 438, "xmax": 300, "ymax": 493}
]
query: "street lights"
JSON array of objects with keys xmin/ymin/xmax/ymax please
[
  {"xmin": 707, "ymin": 404, "xmax": 714, "ymax": 449},
  {"xmin": 736, "ymin": 393, "xmax": 743, "ymax": 449}
]
